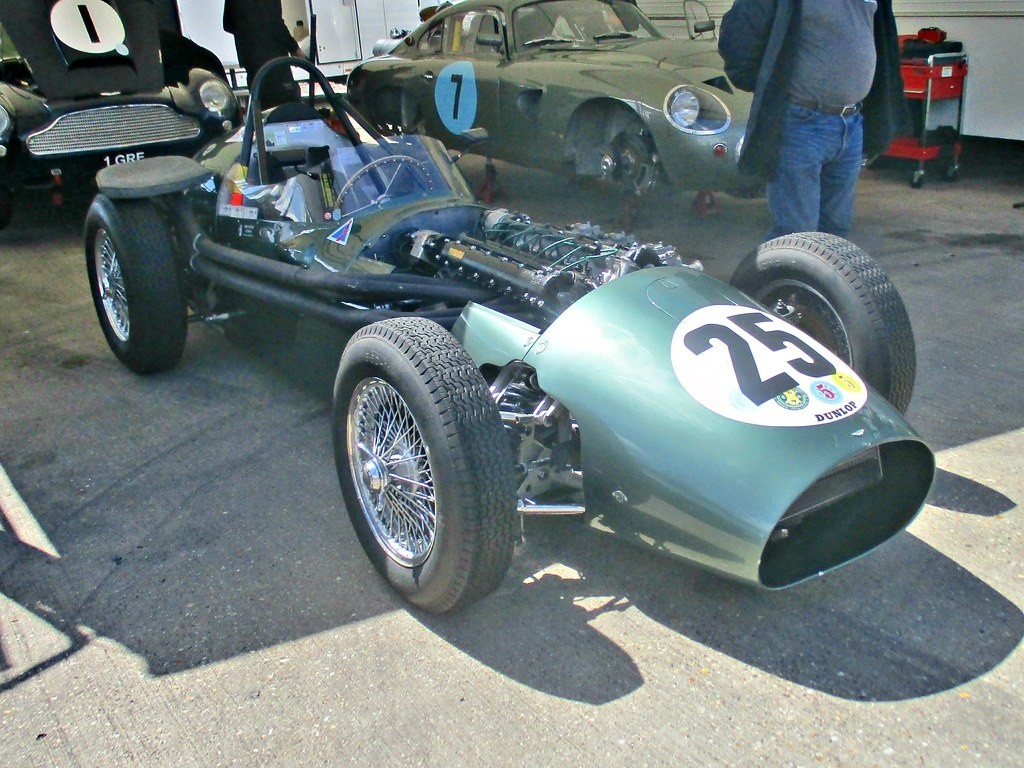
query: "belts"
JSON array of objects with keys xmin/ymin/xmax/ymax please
[{"xmin": 787, "ymin": 94, "xmax": 859, "ymax": 117}]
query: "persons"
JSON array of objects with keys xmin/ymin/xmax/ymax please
[
  {"xmin": 718, "ymin": 0, "xmax": 918, "ymax": 240},
  {"xmin": 575, "ymin": 0, "xmax": 640, "ymax": 36},
  {"xmin": 222, "ymin": 0, "xmax": 307, "ymax": 113}
]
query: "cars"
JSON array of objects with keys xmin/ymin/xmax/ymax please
[
  {"xmin": 346, "ymin": 0, "xmax": 763, "ymax": 214},
  {"xmin": 0, "ymin": 0, "xmax": 245, "ymax": 231}
]
